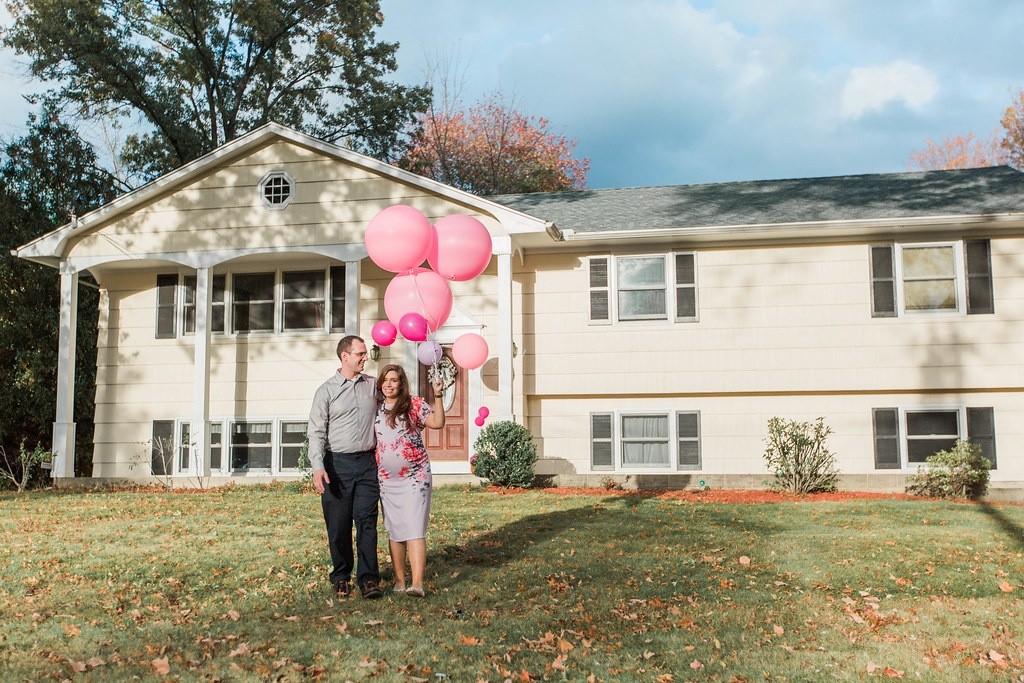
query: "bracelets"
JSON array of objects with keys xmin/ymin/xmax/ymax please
[{"xmin": 434, "ymin": 394, "xmax": 443, "ymax": 398}]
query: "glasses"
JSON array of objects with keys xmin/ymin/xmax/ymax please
[{"xmin": 342, "ymin": 350, "xmax": 367, "ymax": 356}]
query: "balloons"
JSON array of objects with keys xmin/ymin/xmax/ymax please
[
  {"xmin": 399, "ymin": 313, "xmax": 427, "ymax": 341},
  {"xmin": 364, "ymin": 205, "xmax": 432, "ymax": 274},
  {"xmin": 372, "ymin": 320, "xmax": 397, "ymax": 347},
  {"xmin": 418, "ymin": 340, "xmax": 443, "ymax": 366},
  {"xmin": 452, "ymin": 333, "xmax": 489, "ymax": 370},
  {"xmin": 384, "ymin": 267, "xmax": 452, "ymax": 337},
  {"xmin": 475, "ymin": 407, "xmax": 489, "ymax": 426},
  {"xmin": 427, "ymin": 214, "xmax": 492, "ymax": 282}
]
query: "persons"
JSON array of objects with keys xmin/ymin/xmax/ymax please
[
  {"xmin": 372, "ymin": 364, "xmax": 445, "ymax": 597},
  {"xmin": 306, "ymin": 335, "xmax": 385, "ymax": 600}
]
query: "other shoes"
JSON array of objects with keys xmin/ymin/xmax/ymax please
[
  {"xmin": 405, "ymin": 587, "xmax": 425, "ymax": 598},
  {"xmin": 393, "ymin": 584, "xmax": 406, "ymax": 592},
  {"xmin": 360, "ymin": 576, "xmax": 382, "ymax": 598},
  {"xmin": 333, "ymin": 580, "xmax": 351, "ymax": 597}
]
